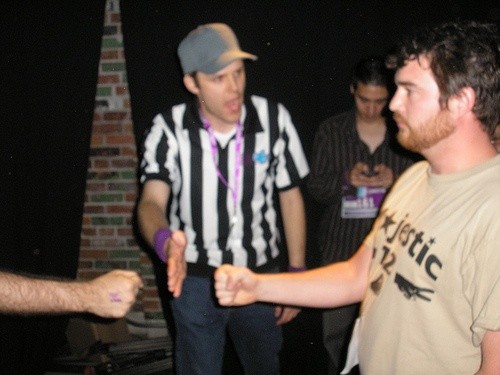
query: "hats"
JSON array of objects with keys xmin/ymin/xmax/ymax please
[{"xmin": 177, "ymin": 22, "xmax": 258, "ymax": 74}]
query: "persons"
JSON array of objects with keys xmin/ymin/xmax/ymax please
[
  {"xmin": 214, "ymin": 20, "xmax": 499, "ymax": 375},
  {"xmin": 137, "ymin": 22, "xmax": 311, "ymax": 375},
  {"xmin": 306, "ymin": 53, "xmax": 500, "ymax": 375},
  {"xmin": 0, "ymin": 268, "xmax": 146, "ymax": 317}
]
